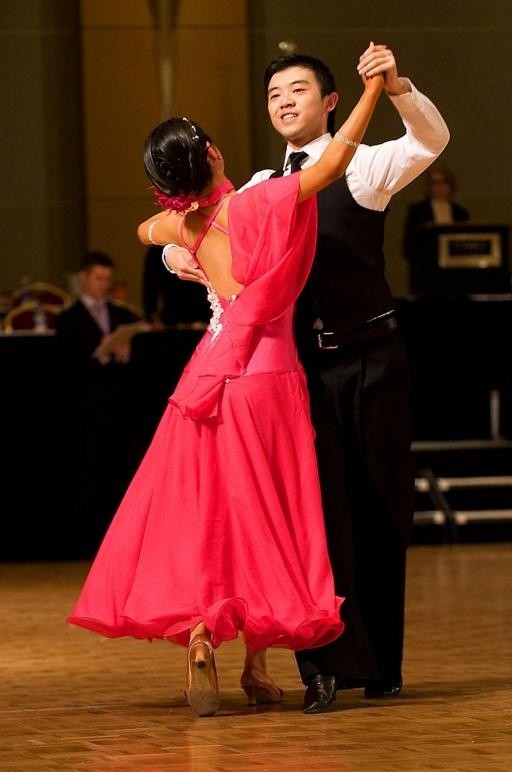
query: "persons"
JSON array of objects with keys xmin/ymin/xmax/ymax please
[
  {"xmin": 137, "ymin": 43, "xmax": 391, "ymax": 713},
  {"xmin": 52, "ymin": 252, "xmax": 148, "ymax": 366},
  {"xmin": 162, "ymin": 42, "xmax": 451, "ymax": 710},
  {"xmin": 400, "ymin": 164, "xmax": 475, "ymax": 291},
  {"xmin": 141, "ymin": 237, "xmax": 208, "ymax": 331}
]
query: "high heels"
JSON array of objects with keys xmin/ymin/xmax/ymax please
[
  {"xmin": 183, "ymin": 632, "xmax": 221, "ymax": 718},
  {"xmin": 239, "ymin": 665, "xmax": 285, "ymax": 707}
]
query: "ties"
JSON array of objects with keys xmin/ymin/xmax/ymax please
[
  {"xmin": 92, "ymin": 302, "xmax": 110, "ymax": 337},
  {"xmin": 288, "ymin": 151, "xmax": 309, "ymax": 175}
]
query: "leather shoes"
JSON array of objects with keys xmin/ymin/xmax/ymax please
[
  {"xmin": 363, "ymin": 673, "xmax": 403, "ymax": 699},
  {"xmin": 302, "ymin": 672, "xmax": 338, "ymax": 714}
]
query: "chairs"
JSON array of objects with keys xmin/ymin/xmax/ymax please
[{"xmin": 4, "ymin": 280, "xmax": 148, "ymax": 336}]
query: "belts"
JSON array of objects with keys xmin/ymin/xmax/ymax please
[{"xmin": 296, "ymin": 307, "xmax": 402, "ymax": 351}]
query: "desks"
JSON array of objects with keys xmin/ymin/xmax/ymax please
[{"xmin": 1, "ymin": 327, "xmax": 208, "ymax": 568}]
